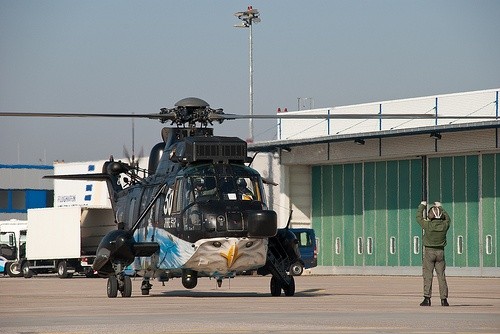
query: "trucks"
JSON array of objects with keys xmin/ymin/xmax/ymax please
[{"xmin": 0, "ymin": 206, "xmax": 117, "ymax": 277}]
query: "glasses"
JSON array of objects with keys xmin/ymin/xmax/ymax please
[
  {"xmin": 195, "ymin": 184, "xmax": 204, "ymax": 187},
  {"xmin": 239, "ymin": 185, "xmax": 246, "ymax": 187}
]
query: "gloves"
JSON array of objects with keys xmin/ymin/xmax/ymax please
[
  {"xmin": 421, "ymin": 200, "xmax": 427, "ymax": 206},
  {"xmin": 434, "ymin": 201, "xmax": 441, "ymax": 207}
]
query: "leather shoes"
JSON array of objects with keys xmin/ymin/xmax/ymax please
[
  {"xmin": 441, "ymin": 298, "xmax": 450, "ymax": 306},
  {"xmin": 420, "ymin": 296, "xmax": 431, "ymax": 305}
]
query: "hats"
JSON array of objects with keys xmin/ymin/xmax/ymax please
[
  {"xmin": 427, "ymin": 206, "xmax": 443, "ymax": 219},
  {"xmin": 194, "ymin": 178, "xmax": 205, "ymax": 184},
  {"xmin": 236, "ymin": 178, "xmax": 247, "ymax": 185}
]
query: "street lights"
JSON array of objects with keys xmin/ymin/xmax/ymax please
[{"xmin": 233, "ymin": 5, "xmax": 261, "ymax": 142}]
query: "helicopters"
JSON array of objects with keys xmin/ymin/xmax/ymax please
[{"xmin": 0, "ymin": 96, "xmax": 500, "ymax": 301}]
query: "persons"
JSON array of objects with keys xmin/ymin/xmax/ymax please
[
  {"xmin": 186, "ymin": 176, "xmax": 217, "ymax": 229},
  {"xmin": 416, "ymin": 200, "xmax": 451, "ymax": 307},
  {"xmin": 234, "ymin": 178, "xmax": 254, "ymax": 200}
]
